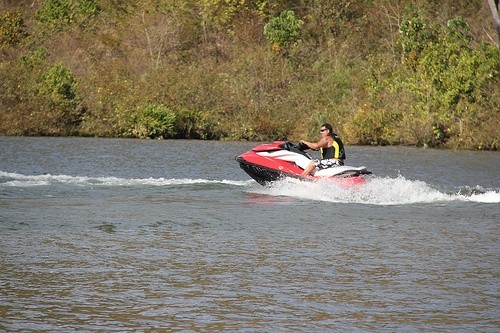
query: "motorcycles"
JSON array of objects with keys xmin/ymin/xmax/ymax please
[{"xmin": 236, "ymin": 139, "xmax": 372, "ymax": 192}]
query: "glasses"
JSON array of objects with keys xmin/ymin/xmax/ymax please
[{"xmin": 321, "ymin": 129, "xmax": 327, "ymax": 132}]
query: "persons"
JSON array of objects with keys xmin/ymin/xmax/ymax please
[{"xmin": 300, "ymin": 123, "xmax": 346, "ymax": 176}]
